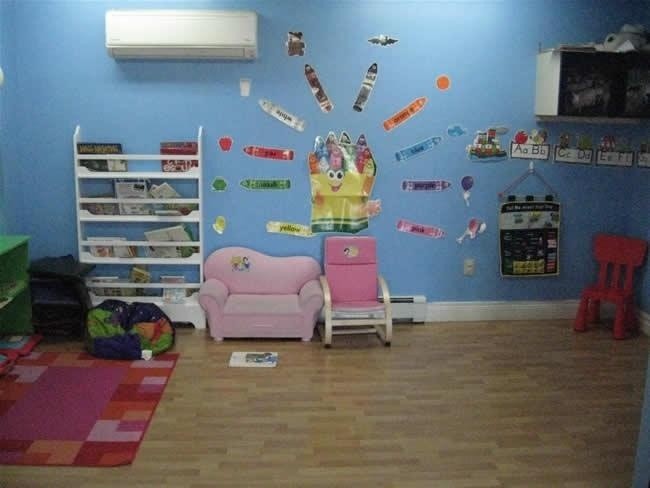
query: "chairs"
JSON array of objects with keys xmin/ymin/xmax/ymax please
[
  {"xmin": 573, "ymin": 233, "xmax": 649, "ymax": 339},
  {"xmin": 200, "ymin": 245, "xmax": 323, "ymax": 343},
  {"xmin": 315, "ymin": 235, "xmax": 395, "ymax": 349}
]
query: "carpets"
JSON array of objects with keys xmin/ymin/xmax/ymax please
[{"xmin": 0, "ymin": 350, "xmax": 181, "ymax": 468}]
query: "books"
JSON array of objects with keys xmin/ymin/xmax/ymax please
[
  {"xmin": 78, "ymin": 141, "xmax": 198, "ymax": 304},
  {"xmin": 228, "ymin": 351, "xmax": 280, "ymax": 368},
  {"xmin": 543, "ymin": 40, "xmax": 596, "ymax": 53}
]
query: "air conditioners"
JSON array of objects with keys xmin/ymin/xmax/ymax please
[{"xmin": 104, "ymin": 9, "xmax": 258, "ymax": 63}]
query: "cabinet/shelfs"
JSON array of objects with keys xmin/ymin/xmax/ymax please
[
  {"xmin": 0, "ymin": 234, "xmax": 33, "ymax": 334},
  {"xmin": 71, "ymin": 124, "xmax": 209, "ymax": 331},
  {"xmin": 535, "ymin": 47, "xmax": 650, "ymax": 122}
]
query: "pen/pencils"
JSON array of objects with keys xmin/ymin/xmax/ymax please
[
  {"xmin": 396, "ymin": 218, "xmax": 449, "ymax": 239},
  {"xmin": 307, "ymin": 131, "xmax": 377, "ymax": 175},
  {"xmin": 265, "ymin": 221, "xmax": 316, "ymax": 238},
  {"xmin": 402, "ymin": 180, "xmax": 452, "ymax": 191},
  {"xmin": 304, "ymin": 63, "xmax": 334, "ymax": 114},
  {"xmin": 259, "ymin": 99, "xmax": 305, "ymax": 132},
  {"xmin": 382, "ymin": 97, "xmax": 428, "ymax": 132},
  {"xmin": 353, "ymin": 63, "xmax": 378, "ymax": 112},
  {"xmin": 395, "ymin": 136, "xmax": 442, "ymax": 162},
  {"xmin": 243, "ymin": 146, "xmax": 294, "ymax": 161},
  {"xmin": 240, "ymin": 178, "xmax": 291, "ymax": 190}
]
